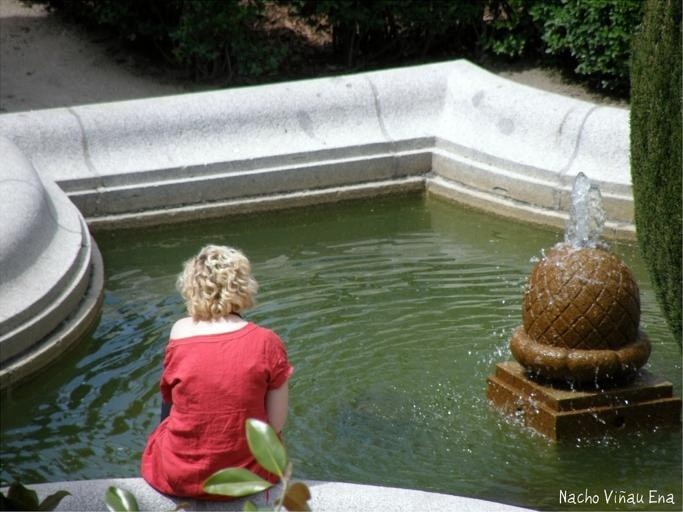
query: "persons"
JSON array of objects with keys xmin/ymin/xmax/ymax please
[{"xmin": 137, "ymin": 245, "xmax": 294, "ymax": 502}]
